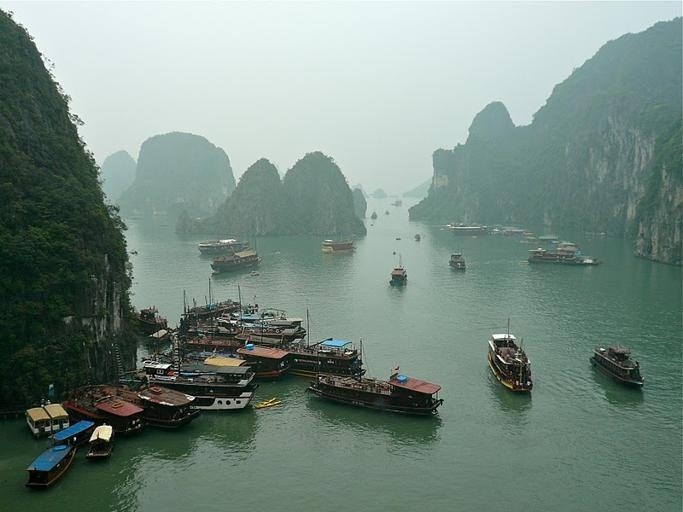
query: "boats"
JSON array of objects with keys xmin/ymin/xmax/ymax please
[
  {"xmin": 415, "ymin": 234, "xmax": 421, "ymax": 242},
  {"xmin": 23, "ymin": 444, "xmax": 78, "ymax": 490},
  {"xmin": 441, "ymin": 222, "xmax": 576, "ymax": 252},
  {"xmin": 25, "ymin": 407, "xmax": 52, "ymax": 439},
  {"xmin": 448, "ymin": 253, "xmax": 467, "ymax": 269},
  {"xmin": 371, "ymin": 211, "xmax": 378, "ymax": 219},
  {"xmin": 43, "ymin": 403, "xmax": 70, "ymax": 433},
  {"xmin": 116, "ymin": 278, "xmax": 310, "ymax": 410},
  {"xmin": 60, "ymin": 384, "xmax": 149, "ymax": 438},
  {"xmin": 526, "ymin": 247, "xmax": 603, "ymax": 266},
  {"xmin": 46, "ymin": 420, "xmax": 96, "ymax": 449},
  {"xmin": 487, "ymin": 317, "xmax": 534, "ymax": 391},
  {"xmin": 321, "ymin": 239, "xmax": 354, "ymax": 254},
  {"xmin": 589, "ymin": 343, "xmax": 645, "ymax": 388},
  {"xmin": 136, "ymin": 307, "xmax": 169, "ymax": 333},
  {"xmin": 196, "ymin": 237, "xmax": 250, "ymax": 256},
  {"xmin": 112, "ymin": 384, "xmax": 201, "ymax": 431},
  {"xmin": 304, "ymin": 337, "xmax": 444, "ymax": 416},
  {"xmin": 85, "ymin": 424, "xmax": 116, "ymax": 459},
  {"xmin": 209, "ymin": 246, "xmax": 264, "ymax": 272},
  {"xmin": 389, "ymin": 265, "xmax": 407, "ymax": 285}
]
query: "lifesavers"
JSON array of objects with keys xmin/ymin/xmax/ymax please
[
  {"xmin": 168, "ymin": 371, "xmax": 175, "ymax": 375},
  {"xmin": 112, "ymin": 402, "xmax": 124, "ymax": 408},
  {"xmin": 150, "ymin": 386, "xmax": 161, "ymax": 395}
]
